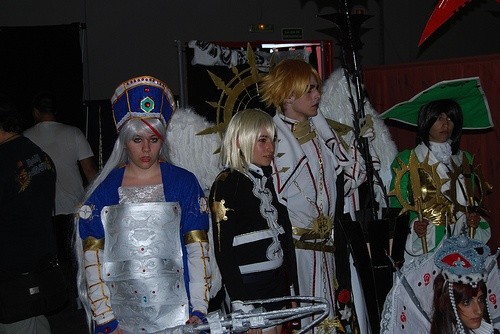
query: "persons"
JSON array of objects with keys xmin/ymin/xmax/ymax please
[
  {"xmin": 389, "ymin": 98, "xmax": 491, "ymax": 274},
  {"xmin": 22, "ymin": 89, "xmax": 100, "ymax": 334},
  {"xmin": 0, "ymin": 95, "xmax": 57, "ymax": 334},
  {"xmin": 209, "ymin": 109, "xmax": 302, "ymax": 334},
  {"xmin": 430, "ymin": 268, "xmax": 495, "ymax": 334},
  {"xmin": 71, "ymin": 75, "xmax": 213, "ymax": 334},
  {"xmin": 257, "ymin": 58, "xmax": 380, "ymax": 334}
]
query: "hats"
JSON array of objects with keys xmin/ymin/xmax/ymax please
[
  {"xmin": 378, "ymin": 76, "xmax": 493, "ymax": 131},
  {"xmin": 111, "ymin": 76, "xmax": 177, "ymax": 132}
]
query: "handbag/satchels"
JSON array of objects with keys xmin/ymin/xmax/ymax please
[{"xmin": 0, "ymin": 258, "xmax": 68, "ymax": 323}]
